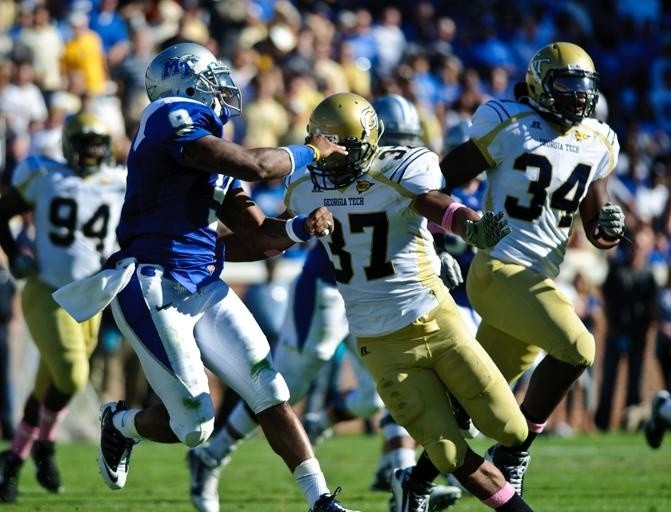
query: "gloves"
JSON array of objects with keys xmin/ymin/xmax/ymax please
[
  {"xmin": 597, "ymin": 202, "xmax": 625, "ymax": 241},
  {"xmin": 465, "ymin": 211, "xmax": 511, "ymax": 249}
]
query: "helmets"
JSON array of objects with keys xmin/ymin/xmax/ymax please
[
  {"xmin": 306, "ymin": 92, "xmax": 386, "ymax": 190},
  {"xmin": 369, "ymin": 92, "xmax": 425, "ymax": 148},
  {"xmin": 144, "ymin": 43, "xmax": 243, "ymax": 120},
  {"xmin": 61, "ymin": 111, "xmax": 111, "ymax": 176},
  {"xmin": 525, "ymin": 41, "xmax": 600, "ymax": 125}
]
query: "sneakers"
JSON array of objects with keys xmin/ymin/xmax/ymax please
[
  {"xmin": 484, "ymin": 444, "xmax": 497, "ymax": 461},
  {"xmin": 302, "ymin": 412, "xmax": 333, "ymax": 450},
  {"xmin": 388, "ymin": 465, "xmax": 429, "ymax": 512},
  {"xmin": 424, "ymin": 479, "xmax": 461, "ymax": 512},
  {"xmin": 645, "ymin": 390, "xmax": 671, "ymax": 448},
  {"xmin": 493, "ymin": 446, "xmax": 530, "ymax": 499},
  {"xmin": 31, "ymin": 440, "xmax": 60, "ymax": 494},
  {"xmin": 0, "ymin": 450, "xmax": 25, "ymax": 503},
  {"xmin": 372, "ymin": 455, "xmax": 417, "ymax": 490},
  {"xmin": 307, "ymin": 486, "xmax": 361, "ymax": 512},
  {"xmin": 98, "ymin": 400, "xmax": 141, "ymax": 491},
  {"xmin": 188, "ymin": 446, "xmax": 231, "ymax": 512}
]
